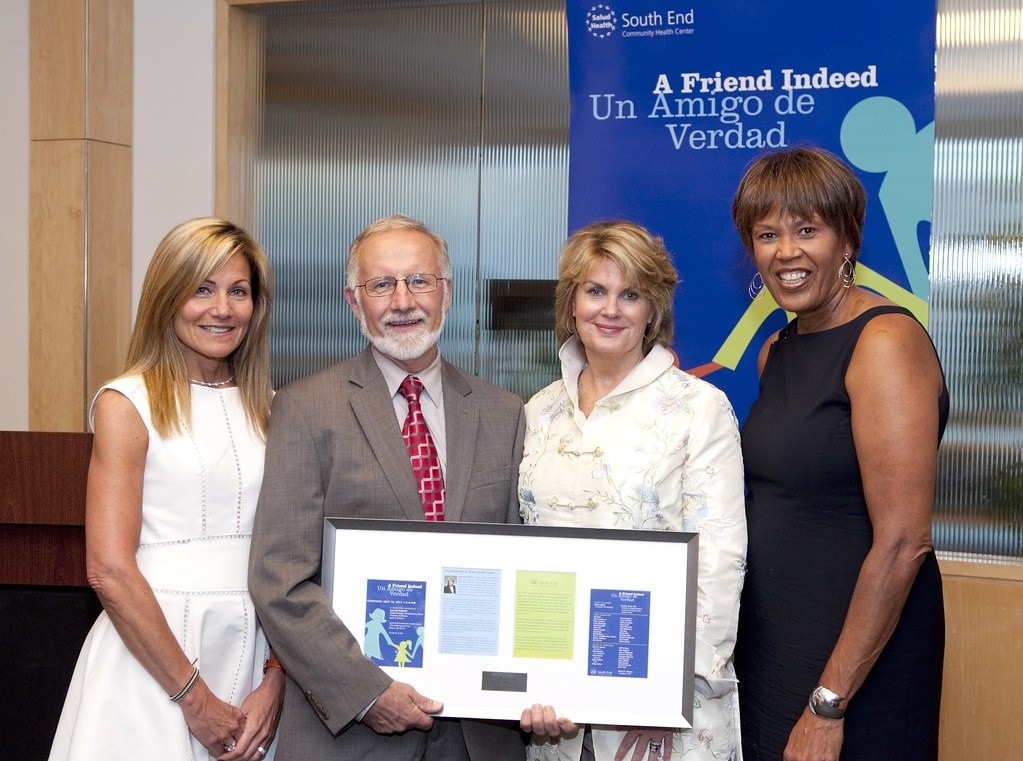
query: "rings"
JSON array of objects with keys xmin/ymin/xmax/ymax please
[
  {"xmin": 258, "ymin": 747, "xmax": 268, "ymax": 756},
  {"xmin": 650, "ymin": 741, "xmax": 662, "ymax": 753},
  {"xmin": 224, "ymin": 741, "xmax": 236, "ymax": 751}
]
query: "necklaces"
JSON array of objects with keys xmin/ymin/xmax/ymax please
[{"xmin": 188, "ymin": 374, "xmax": 235, "ymax": 389}]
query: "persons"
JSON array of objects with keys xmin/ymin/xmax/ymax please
[
  {"xmin": 45, "ymin": 213, "xmax": 283, "ymax": 761},
  {"xmin": 444, "ymin": 577, "xmax": 455, "ymax": 593},
  {"xmin": 725, "ymin": 146, "xmax": 949, "ymax": 760},
  {"xmin": 246, "ymin": 210, "xmax": 581, "ymax": 759},
  {"xmin": 516, "ymin": 219, "xmax": 748, "ymax": 759}
]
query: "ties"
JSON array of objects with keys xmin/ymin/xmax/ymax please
[{"xmin": 399, "ymin": 376, "xmax": 446, "ymax": 521}]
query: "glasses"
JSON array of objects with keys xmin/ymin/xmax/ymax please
[{"xmin": 354, "ymin": 272, "xmax": 448, "ymax": 298}]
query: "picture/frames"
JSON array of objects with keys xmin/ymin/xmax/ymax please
[{"xmin": 321, "ymin": 513, "xmax": 701, "ymax": 735}]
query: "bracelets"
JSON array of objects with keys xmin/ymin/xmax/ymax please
[
  {"xmin": 806, "ymin": 684, "xmax": 848, "ymax": 720},
  {"xmin": 170, "ymin": 658, "xmax": 201, "ymax": 703},
  {"xmin": 263, "ymin": 657, "xmax": 285, "ymax": 674}
]
